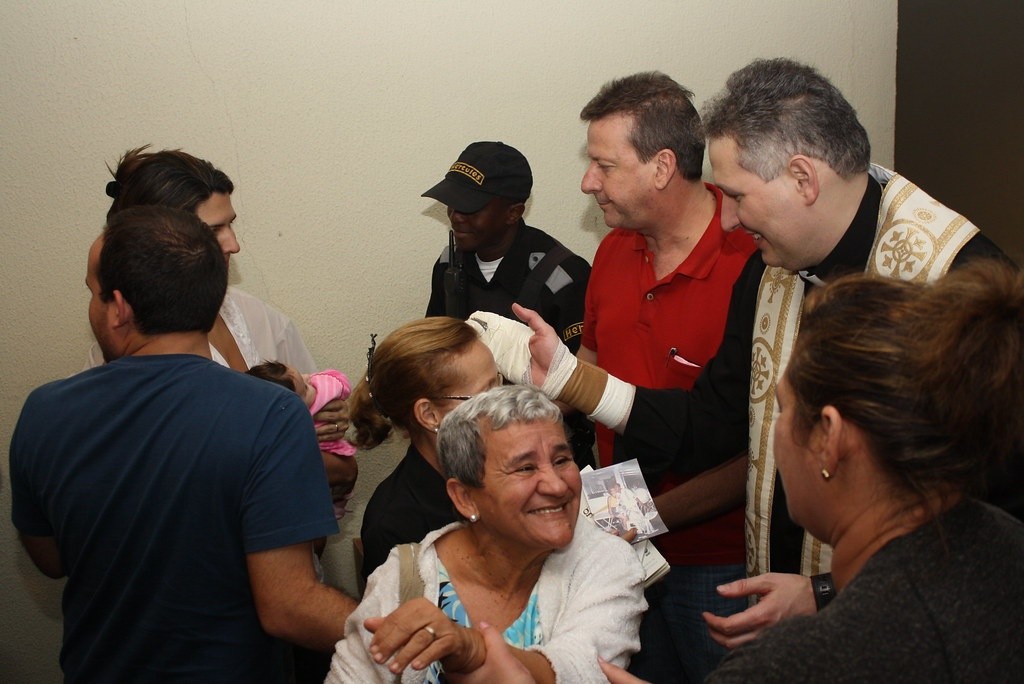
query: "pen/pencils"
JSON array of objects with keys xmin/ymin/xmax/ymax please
[{"xmin": 665, "ymin": 347, "xmax": 678, "ymax": 368}]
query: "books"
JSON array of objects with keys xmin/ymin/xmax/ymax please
[{"xmin": 580, "ymin": 465, "xmax": 670, "ymax": 587}]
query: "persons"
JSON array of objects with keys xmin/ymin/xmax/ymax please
[
  {"xmin": 547, "ymin": 71, "xmax": 757, "ymax": 684},
  {"xmin": 463, "ymin": 60, "xmax": 986, "ymax": 651},
  {"xmin": 324, "ymin": 385, "xmax": 648, "ymax": 683},
  {"xmin": 8, "ymin": 199, "xmax": 358, "ymax": 684},
  {"xmin": 422, "ymin": 140, "xmax": 593, "ymax": 466},
  {"xmin": 437, "ymin": 263, "xmax": 1024, "ymax": 684},
  {"xmin": 350, "ymin": 315, "xmax": 501, "ymax": 577},
  {"xmin": 109, "ymin": 146, "xmax": 358, "ymax": 560},
  {"xmin": 608, "ymin": 483, "xmax": 653, "ymax": 535},
  {"xmin": 250, "ymin": 361, "xmax": 356, "ymax": 519}
]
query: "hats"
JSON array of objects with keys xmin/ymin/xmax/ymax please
[{"xmin": 422, "ymin": 142, "xmax": 533, "ymax": 214}]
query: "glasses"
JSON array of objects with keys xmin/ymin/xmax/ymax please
[{"xmin": 429, "ymin": 374, "xmax": 504, "ymax": 401}]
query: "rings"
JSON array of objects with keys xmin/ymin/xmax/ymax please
[
  {"xmin": 335, "ymin": 423, "xmax": 338, "ymax": 432},
  {"xmin": 424, "ymin": 626, "xmax": 437, "ymax": 640}
]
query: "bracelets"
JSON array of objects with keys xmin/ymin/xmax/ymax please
[{"xmin": 810, "ymin": 571, "xmax": 838, "ymax": 612}]
